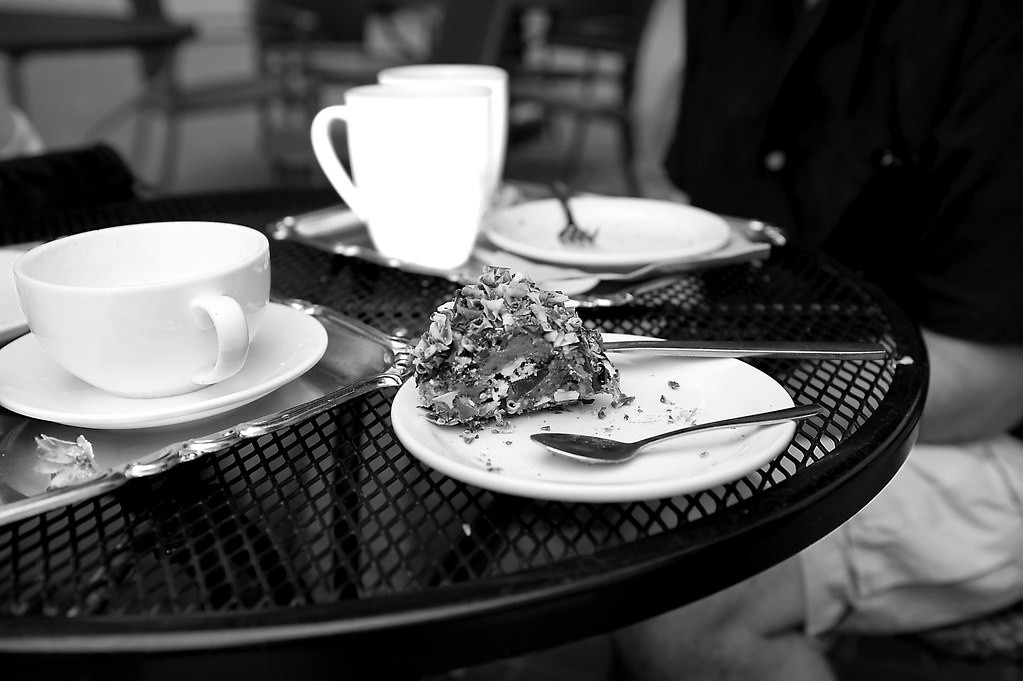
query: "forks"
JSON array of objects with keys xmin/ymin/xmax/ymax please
[{"xmin": 552, "ymin": 188, "xmax": 598, "ymax": 242}]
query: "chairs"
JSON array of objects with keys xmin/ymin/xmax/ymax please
[
  {"xmin": 510, "ymin": 0, "xmax": 655, "ymax": 196},
  {"xmin": 275, "ymin": 0, "xmax": 522, "ymax": 185}
]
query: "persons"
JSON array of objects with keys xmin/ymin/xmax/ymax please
[{"xmin": 466, "ymin": 2, "xmax": 1023, "ymax": 681}]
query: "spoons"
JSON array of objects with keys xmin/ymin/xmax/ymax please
[{"xmin": 529, "ymin": 402, "xmax": 823, "ymax": 464}]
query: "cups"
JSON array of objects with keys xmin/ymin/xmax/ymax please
[
  {"xmin": 12, "ymin": 220, "xmax": 270, "ymax": 398},
  {"xmin": 311, "ymin": 63, "xmax": 511, "ymax": 267}
]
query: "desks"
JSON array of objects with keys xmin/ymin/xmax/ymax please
[{"xmin": 0, "ymin": 11, "xmax": 198, "ymax": 192}]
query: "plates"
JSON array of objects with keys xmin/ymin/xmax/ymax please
[
  {"xmin": 490, "ymin": 193, "xmax": 732, "ymax": 268},
  {"xmin": 0, "ymin": 302, "xmax": 329, "ymax": 445},
  {"xmin": 387, "ymin": 327, "xmax": 799, "ymax": 501}
]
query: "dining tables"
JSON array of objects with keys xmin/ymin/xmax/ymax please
[{"xmin": 1, "ymin": 182, "xmax": 940, "ymax": 679}]
